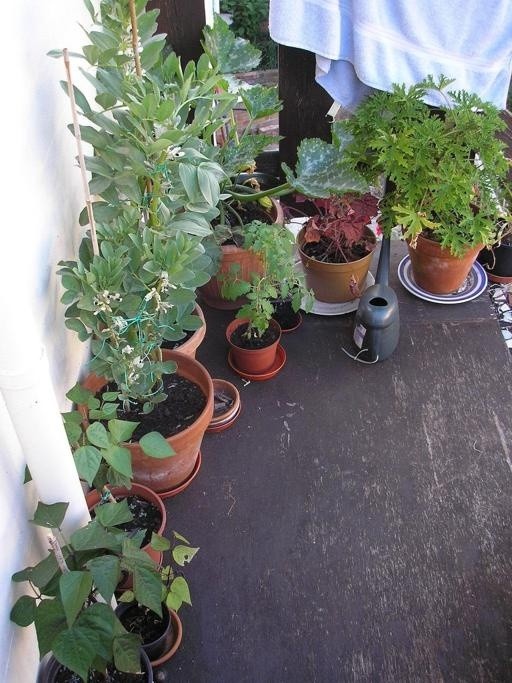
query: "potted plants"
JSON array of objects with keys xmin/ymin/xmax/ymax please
[
  {"xmin": 337, "ymin": 70, "xmax": 509, "ymax": 295},
  {"xmin": 57, "ymin": 210, "xmax": 213, "ymax": 493},
  {"xmin": 219, "ymin": 217, "xmax": 316, "ymax": 373},
  {"xmin": 10, "ymin": 483, "xmax": 184, "ymax": 683},
  {"xmin": 292, "ymin": 194, "xmax": 378, "ymax": 306},
  {"xmin": 87, "ymin": 4, "xmax": 284, "ymax": 355}
]
